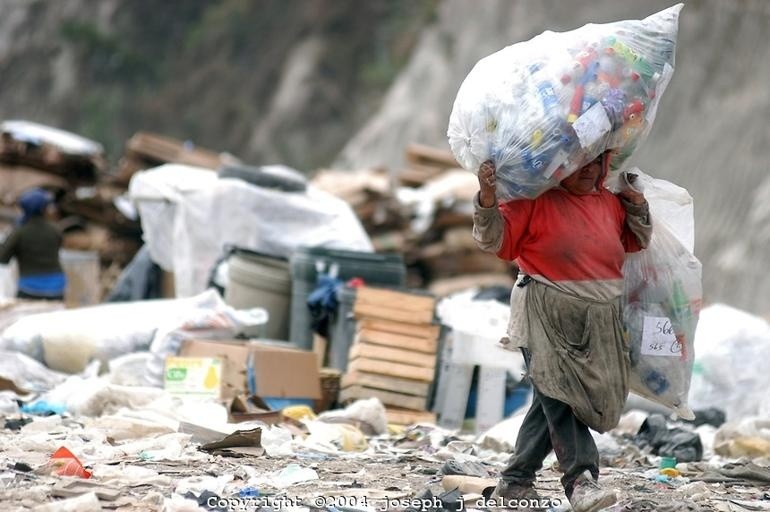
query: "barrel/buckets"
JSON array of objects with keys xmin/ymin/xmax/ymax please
[{"xmin": 225, "ymin": 247, "xmax": 436, "ymax": 373}]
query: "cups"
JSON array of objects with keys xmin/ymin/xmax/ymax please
[{"xmin": 660, "ymin": 454, "xmax": 676, "ymax": 469}]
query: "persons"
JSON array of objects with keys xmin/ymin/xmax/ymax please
[
  {"xmin": 0, "ymin": 189, "xmax": 67, "ymax": 301},
  {"xmin": 470, "ymin": 150, "xmax": 654, "ymax": 512}
]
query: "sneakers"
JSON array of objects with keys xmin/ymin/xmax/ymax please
[
  {"xmin": 570, "ymin": 470, "xmax": 617, "ymax": 512},
  {"xmin": 490, "ymin": 480, "xmax": 546, "ymax": 512}
]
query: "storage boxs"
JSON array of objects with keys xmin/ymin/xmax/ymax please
[
  {"xmin": 179, "ymin": 338, "xmax": 320, "ymax": 409},
  {"xmin": 161, "ymin": 355, "xmax": 240, "ymax": 406}
]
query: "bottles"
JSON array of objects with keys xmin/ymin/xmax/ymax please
[
  {"xmin": 450, "ymin": 18, "xmax": 679, "ymax": 204},
  {"xmin": 625, "ymin": 252, "xmax": 702, "ymax": 411}
]
